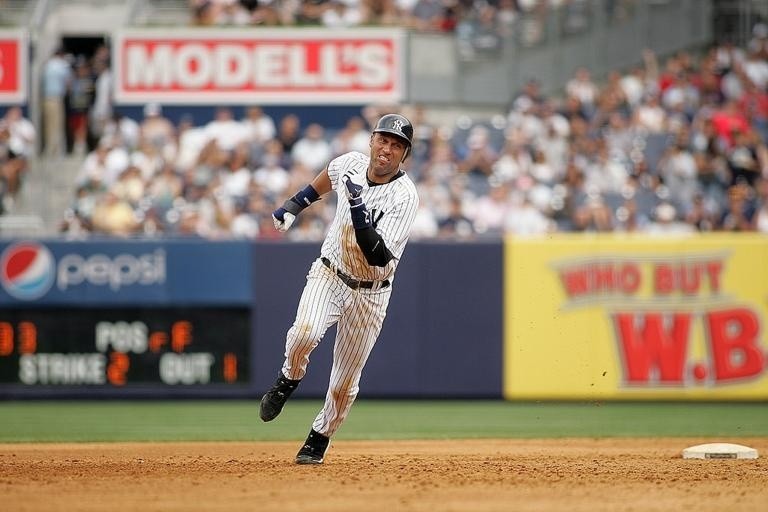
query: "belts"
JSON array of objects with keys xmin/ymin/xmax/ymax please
[{"xmin": 322, "ymin": 258, "xmax": 389, "ymax": 289}]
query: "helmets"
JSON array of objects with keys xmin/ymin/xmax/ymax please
[{"xmin": 372, "ymin": 113, "xmax": 413, "ymax": 163}]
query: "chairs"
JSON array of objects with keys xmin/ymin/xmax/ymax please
[{"xmin": 435, "ymin": 90, "xmax": 768, "ymax": 228}]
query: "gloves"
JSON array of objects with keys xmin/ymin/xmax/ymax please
[
  {"xmin": 271, "ymin": 199, "xmax": 302, "ymax": 233},
  {"xmin": 341, "ymin": 167, "xmax": 366, "ymax": 208}
]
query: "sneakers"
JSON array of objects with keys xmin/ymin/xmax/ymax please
[
  {"xmin": 295, "ymin": 427, "xmax": 330, "ymax": 464},
  {"xmin": 258, "ymin": 370, "xmax": 300, "ymax": 423}
]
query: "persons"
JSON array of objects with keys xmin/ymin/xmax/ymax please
[
  {"xmin": 258, "ymin": 112, "xmax": 421, "ymax": 466},
  {"xmin": 41, "ymin": 44, "xmax": 76, "ymax": 159},
  {"xmin": 58, "ymin": 2, "xmax": 768, "ymax": 239},
  {"xmin": 0, "ymin": 105, "xmax": 35, "ymax": 213}
]
